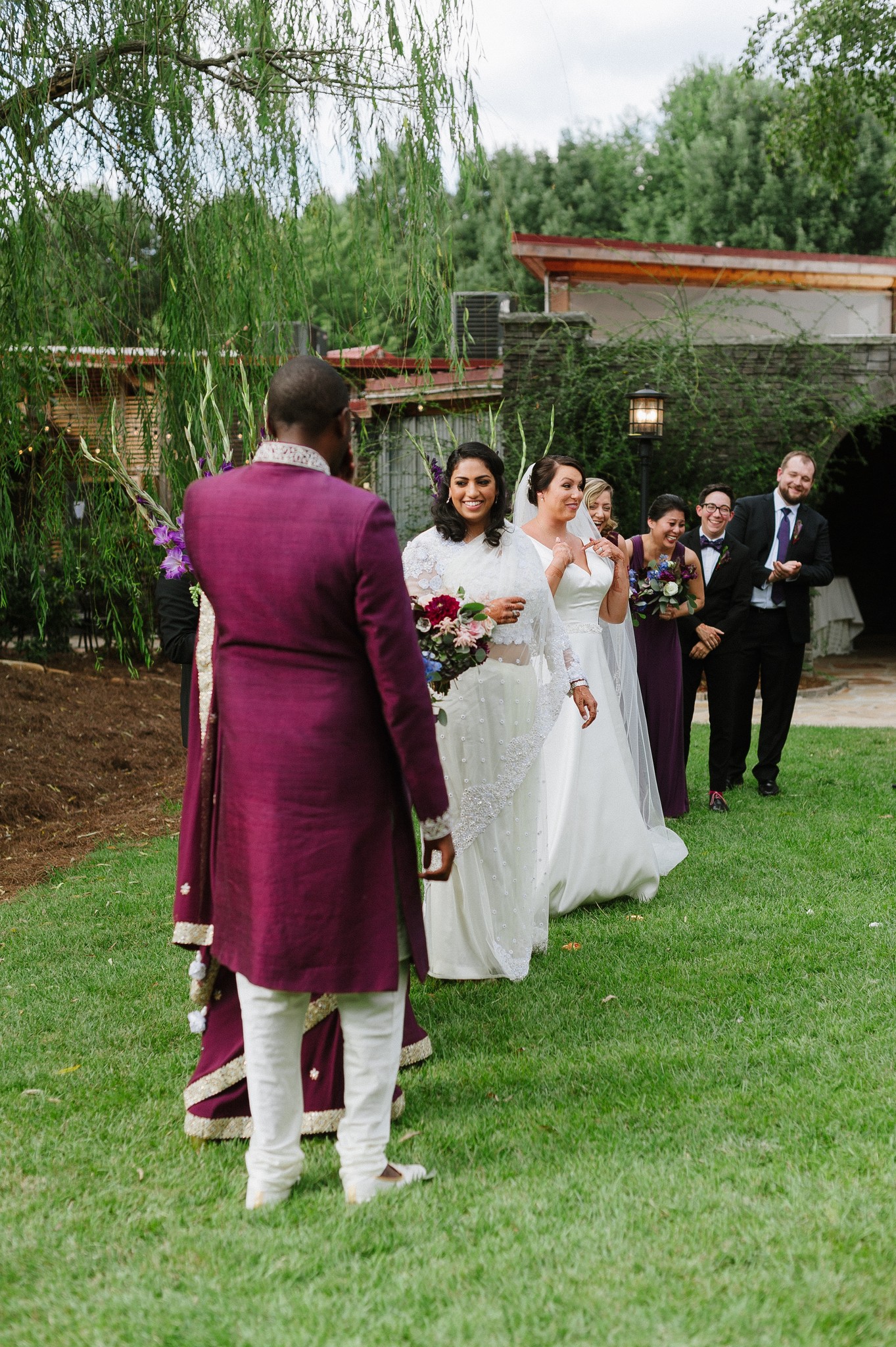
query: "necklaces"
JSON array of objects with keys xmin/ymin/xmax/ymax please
[
  {"xmin": 466, "ymin": 530, "xmax": 474, "ymax": 539},
  {"xmin": 537, "ymin": 520, "xmax": 567, "ymax": 543}
]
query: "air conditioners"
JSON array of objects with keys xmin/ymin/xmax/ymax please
[{"xmin": 448, "ymin": 292, "xmax": 518, "ymax": 357}]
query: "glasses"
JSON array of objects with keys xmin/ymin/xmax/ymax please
[{"xmin": 698, "ymin": 502, "xmax": 731, "ymax": 515}]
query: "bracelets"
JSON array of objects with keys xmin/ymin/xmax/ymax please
[{"xmin": 572, "ymin": 680, "xmax": 589, "ymax": 689}]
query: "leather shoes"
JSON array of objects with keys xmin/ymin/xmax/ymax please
[
  {"xmin": 709, "ymin": 790, "xmax": 725, "ymax": 813},
  {"xmin": 758, "ymin": 780, "xmax": 779, "ymax": 797},
  {"xmin": 351, "ymin": 1163, "xmax": 429, "ymax": 1205}
]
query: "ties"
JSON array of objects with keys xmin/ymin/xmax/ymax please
[{"xmin": 771, "ymin": 508, "xmax": 793, "ymax": 604}]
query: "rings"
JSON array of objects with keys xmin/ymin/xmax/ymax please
[
  {"xmin": 779, "ymin": 574, "xmax": 782, "ymax": 577},
  {"xmin": 664, "ymin": 611, "xmax": 665, "ymax": 615},
  {"xmin": 699, "ymin": 654, "xmax": 702, "ymax": 656},
  {"xmin": 513, "ymin": 612, "xmax": 517, "ymax": 618},
  {"xmin": 599, "ymin": 547, "xmax": 603, "ymax": 553}
]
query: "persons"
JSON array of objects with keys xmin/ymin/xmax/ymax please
[
  {"xmin": 514, "ymin": 455, "xmax": 684, "ymax": 921},
  {"xmin": 585, "ymin": 477, "xmax": 626, "ymax": 566},
  {"xmin": 625, "ymin": 494, "xmax": 705, "ymax": 818},
  {"xmin": 403, "ymin": 441, "xmax": 598, "ymax": 980},
  {"xmin": 678, "ymin": 483, "xmax": 752, "ymax": 814},
  {"xmin": 174, "ymin": 354, "xmax": 456, "ymax": 1207},
  {"xmin": 727, "ymin": 450, "xmax": 835, "ymax": 796}
]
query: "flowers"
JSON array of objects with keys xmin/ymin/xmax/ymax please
[
  {"xmin": 716, "ymin": 547, "xmax": 731, "ymax": 572},
  {"xmin": 793, "ymin": 519, "xmax": 803, "ymax": 543},
  {"xmin": 625, "ymin": 551, "xmax": 700, "ymax": 629},
  {"xmin": 410, "ymin": 585, "xmax": 505, "ymax": 728}
]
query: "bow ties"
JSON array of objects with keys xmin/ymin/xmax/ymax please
[{"xmin": 700, "ymin": 534, "xmax": 726, "ymax": 553}]
